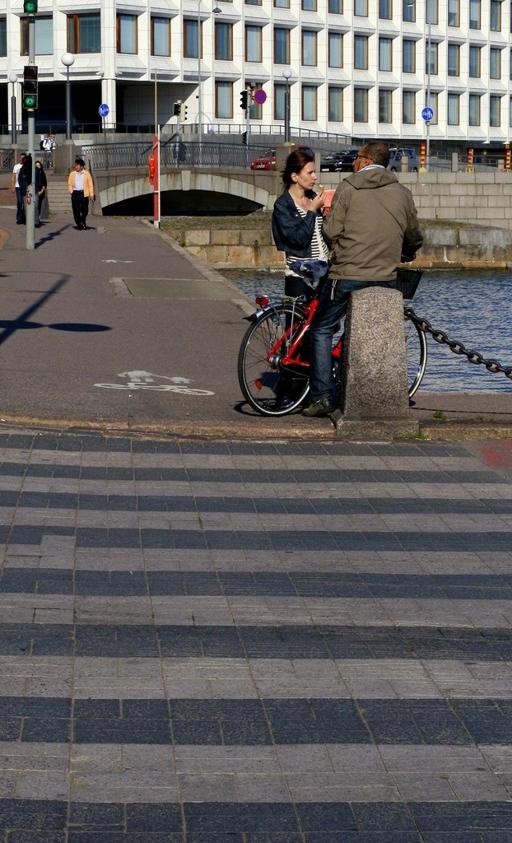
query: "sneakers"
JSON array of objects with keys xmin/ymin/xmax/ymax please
[{"xmin": 303, "ymin": 397, "xmax": 335, "ymax": 416}]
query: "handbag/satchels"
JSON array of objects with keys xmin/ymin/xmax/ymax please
[
  {"xmin": 399, "ymin": 227, "xmax": 424, "ymax": 263},
  {"xmin": 289, "ymin": 259, "xmax": 328, "ymax": 279}
]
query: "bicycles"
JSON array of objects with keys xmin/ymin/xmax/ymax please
[{"xmin": 238, "ymin": 269, "xmax": 427, "ymax": 417}]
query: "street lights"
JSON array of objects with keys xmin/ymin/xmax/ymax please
[
  {"xmin": 198, "ymin": 0, "xmax": 222, "ymax": 163},
  {"xmin": 9, "ymin": 74, "xmax": 17, "ymax": 144},
  {"xmin": 283, "ymin": 69, "xmax": 291, "ymax": 142},
  {"xmin": 61, "ymin": 54, "xmax": 74, "ymax": 139}
]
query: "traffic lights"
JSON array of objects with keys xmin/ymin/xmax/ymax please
[
  {"xmin": 23, "ymin": 66, "xmax": 38, "ymax": 109},
  {"xmin": 23, "ymin": 0, "xmax": 38, "ymax": 13}
]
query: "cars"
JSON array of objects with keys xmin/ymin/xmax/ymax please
[
  {"xmin": 251, "ymin": 150, "xmax": 276, "ymax": 171},
  {"xmin": 320, "ymin": 150, "xmax": 358, "ymax": 172}
]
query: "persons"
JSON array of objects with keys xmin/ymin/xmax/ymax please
[
  {"xmin": 11, "ymin": 152, "xmax": 49, "ymax": 227},
  {"xmin": 68, "ymin": 158, "xmax": 95, "ymax": 231},
  {"xmin": 42, "ymin": 133, "xmax": 52, "ymax": 170},
  {"xmin": 271, "ymin": 149, "xmax": 331, "ymax": 410},
  {"xmin": 297, "ymin": 140, "xmax": 422, "ymax": 417},
  {"xmin": 49, "ymin": 134, "xmax": 56, "ymax": 168}
]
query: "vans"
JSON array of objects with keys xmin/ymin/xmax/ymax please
[{"xmin": 386, "ymin": 148, "xmax": 419, "ymax": 172}]
